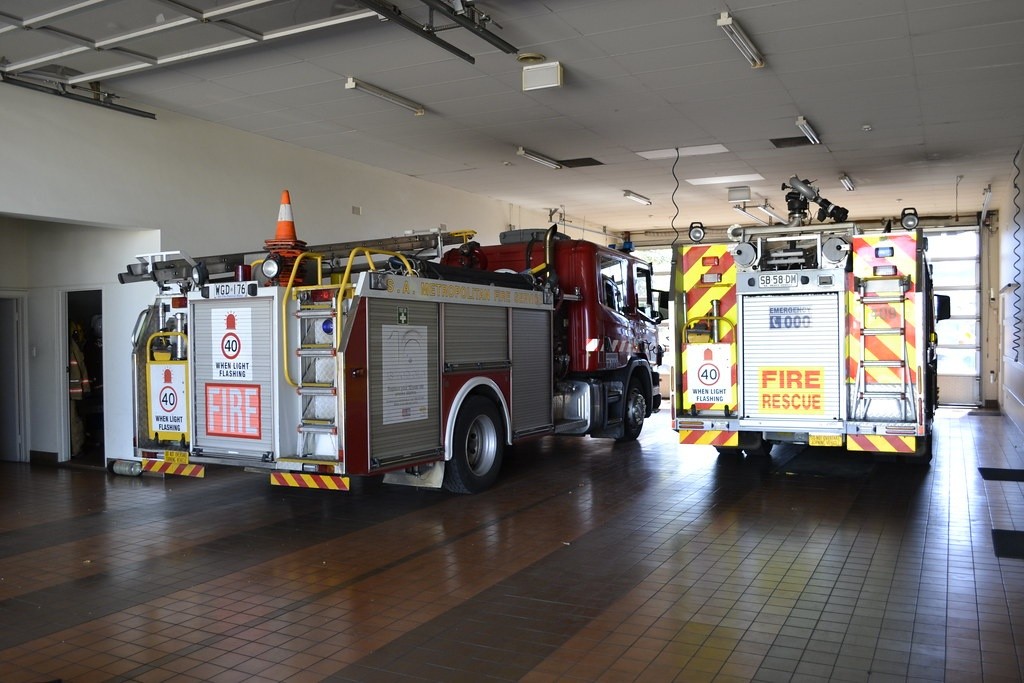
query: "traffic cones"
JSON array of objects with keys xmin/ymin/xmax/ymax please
[{"xmin": 262, "ymin": 189, "xmax": 308, "ymax": 286}]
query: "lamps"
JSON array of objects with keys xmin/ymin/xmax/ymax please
[
  {"xmin": 623, "ymin": 190, "xmax": 651, "ymax": 206},
  {"xmin": 716, "ymin": 12, "xmax": 767, "ymax": 69},
  {"xmin": 345, "ymin": 77, "xmax": 425, "ymax": 117},
  {"xmin": 837, "ymin": 172, "xmax": 856, "ymax": 191},
  {"xmin": 515, "ymin": 147, "xmax": 562, "ymax": 170},
  {"xmin": 794, "ymin": 115, "xmax": 822, "ymax": 145},
  {"xmin": 981, "ymin": 184, "xmax": 993, "ymax": 222},
  {"xmin": 522, "ymin": 61, "xmax": 563, "ymax": 91}
]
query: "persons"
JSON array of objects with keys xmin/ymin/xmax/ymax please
[{"xmin": 69, "ymin": 314, "xmax": 104, "ymax": 460}]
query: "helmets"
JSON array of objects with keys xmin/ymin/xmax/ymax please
[{"xmin": 91, "ymin": 315, "xmax": 101, "ymax": 337}]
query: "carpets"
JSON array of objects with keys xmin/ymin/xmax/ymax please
[
  {"xmin": 978, "ymin": 467, "xmax": 1024, "ymax": 482},
  {"xmin": 968, "ymin": 412, "xmax": 1001, "ymax": 416},
  {"xmin": 991, "ymin": 529, "xmax": 1024, "ymax": 559}
]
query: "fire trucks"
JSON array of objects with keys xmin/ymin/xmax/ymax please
[
  {"xmin": 667, "ymin": 174, "xmax": 953, "ymax": 477},
  {"xmin": 115, "ymin": 184, "xmax": 671, "ymax": 502}
]
